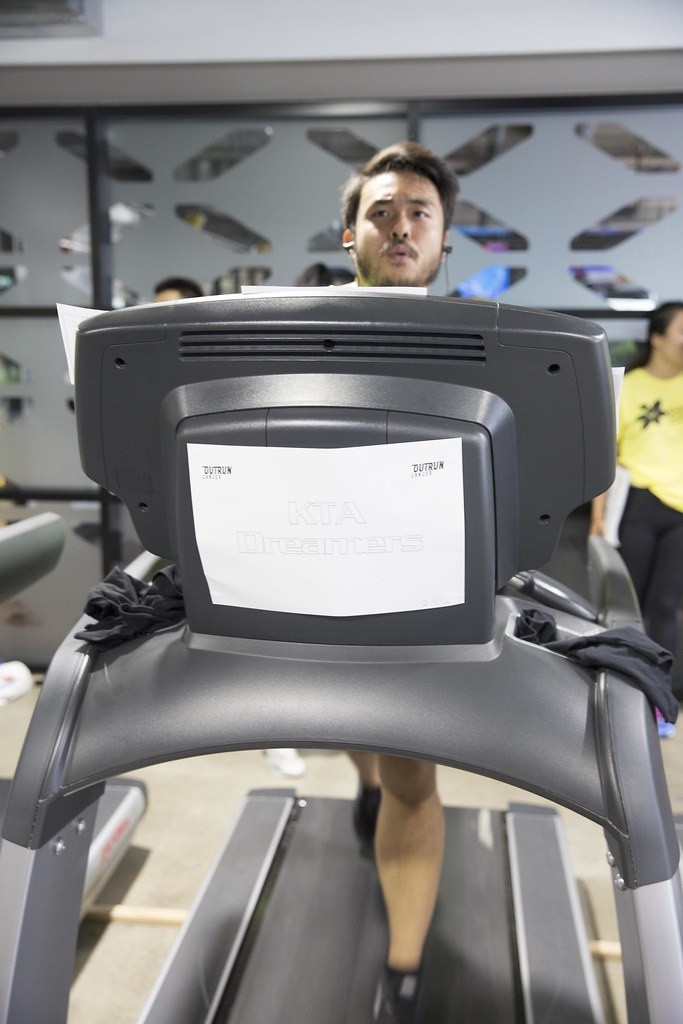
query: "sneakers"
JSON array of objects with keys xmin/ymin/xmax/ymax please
[
  {"xmin": 372, "ymin": 954, "xmax": 427, "ymax": 1024},
  {"xmin": 351, "ymin": 783, "xmax": 379, "ymax": 860}
]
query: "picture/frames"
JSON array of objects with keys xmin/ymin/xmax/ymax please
[{"xmin": 2, "ymin": 1, "xmax": 101, "ymax": 39}]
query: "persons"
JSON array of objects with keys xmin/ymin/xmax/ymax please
[
  {"xmin": 589, "ymin": 300, "xmax": 682, "ymax": 736},
  {"xmin": 336, "ymin": 140, "xmax": 466, "ymax": 1024},
  {"xmin": 156, "ymin": 278, "xmax": 203, "ymax": 306}
]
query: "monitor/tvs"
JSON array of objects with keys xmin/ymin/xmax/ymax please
[{"xmin": 75, "ymin": 288, "xmax": 617, "ymax": 648}]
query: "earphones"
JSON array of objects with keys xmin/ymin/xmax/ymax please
[
  {"xmin": 343, "ymin": 241, "xmax": 355, "ymax": 252},
  {"xmin": 443, "ymin": 244, "xmax": 453, "ymax": 255}
]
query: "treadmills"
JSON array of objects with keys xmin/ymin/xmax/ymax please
[
  {"xmin": 0, "ymin": 288, "xmax": 683, "ymax": 1023},
  {"xmin": 0, "ymin": 509, "xmax": 149, "ymax": 940}
]
